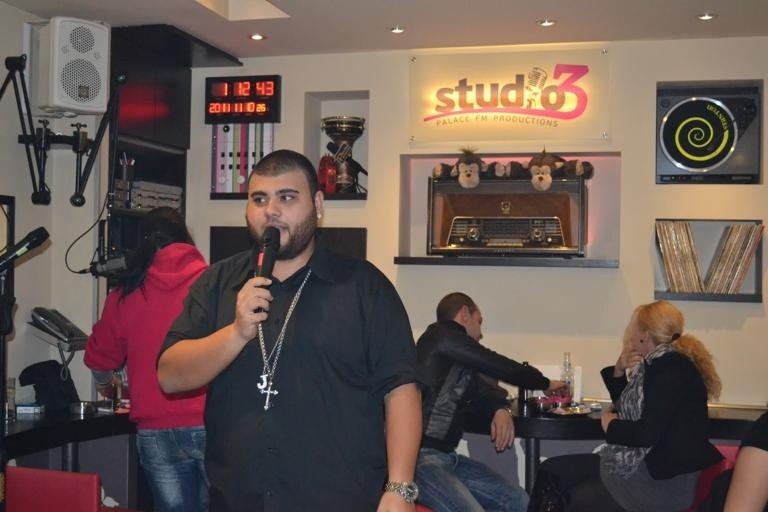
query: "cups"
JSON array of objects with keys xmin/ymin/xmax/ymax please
[{"xmin": 6, "ymin": 376, "xmax": 15, "ymax": 423}]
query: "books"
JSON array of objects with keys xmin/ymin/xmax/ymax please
[
  {"xmin": 213, "ymin": 122, "xmax": 280, "ymax": 198},
  {"xmin": 651, "ymin": 220, "xmax": 766, "ymax": 294}
]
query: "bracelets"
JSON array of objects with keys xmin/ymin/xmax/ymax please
[{"xmin": 504, "ymin": 406, "xmax": 512, "ymax": 416}]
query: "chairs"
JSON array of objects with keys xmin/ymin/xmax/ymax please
[
  {"xmin": 5, "ymin": 466, "xmax": 135, "ymax": 512},
  {"xmin": 673, "ymin": 446, "xmax": 738, "ymax": 512}
]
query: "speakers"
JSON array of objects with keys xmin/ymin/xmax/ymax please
[{"xmin": 34, "ymin": 15, "xmax": 111, "ymax": 119}]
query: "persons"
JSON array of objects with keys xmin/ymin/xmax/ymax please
[
  {"xmin": 414, "ymin": 292, "xmax": 574, "ymax": 511},
  {"xmin": 720, "ymin": 411, "xmax": 768, "ymax": 511},
  {"xmin": 155, "ymin": 149, "xmax": 425, "ymax": 510},
  {"xmin": 527, "ymin": 299, "xmax": 724, "ymax": 512},
  {"xmin": 85, "ymin": 208, "xmax": 212, "ymax": 512}
]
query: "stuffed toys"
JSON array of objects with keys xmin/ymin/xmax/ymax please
[
  {"xmin": 505, "ymin": 147, "xmax": 594, "ymax": 190},
  {"xmin": 432, "ymin": 145, "xmax": 509, "ymax": 189}
]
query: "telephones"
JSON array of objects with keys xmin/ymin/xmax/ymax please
[{"xmin": 26, "ymin": 307, "xmax": 90, "ymax": 351}]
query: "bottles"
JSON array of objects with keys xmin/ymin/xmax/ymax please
[
  {"xmin": 518, "ymin": 362, "xmax": 533, "ymax": 404},
  {"xmin": 563, "ymin": 352, "xmax": 573, "ymax": 404}
]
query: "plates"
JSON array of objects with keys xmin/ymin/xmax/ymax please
[{"xmin": 547, "ymin": 406, "xmax": 594, "ymax": 416}]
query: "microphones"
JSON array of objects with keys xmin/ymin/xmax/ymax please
[
  {"xmin": 252, "ymin": 224, "xmax": 282, "ymax": 321},
  {"xmin": 86, "ymin": 251, "xmax": 145, "ymax": 278},
  {"xmin": 0, "ymin": 226, "xmax": 51, "ymax": 276}
]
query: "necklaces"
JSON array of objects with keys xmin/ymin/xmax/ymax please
[{"xmin": 248, "ymin": 270, "xmax": 312, "ymax": 412}]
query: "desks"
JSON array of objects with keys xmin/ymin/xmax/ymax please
[
  {"xmin": 0, "ymin": 400, "xmax": 135, "ymax": 472},
  {"xmin": 489, "ymin": 400, "xmax": 613, "ymax": 494}
]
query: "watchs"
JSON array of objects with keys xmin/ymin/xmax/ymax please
[{"xmin": 380, "ymin": 479, "xmax": 421, "ymax": 502}]
